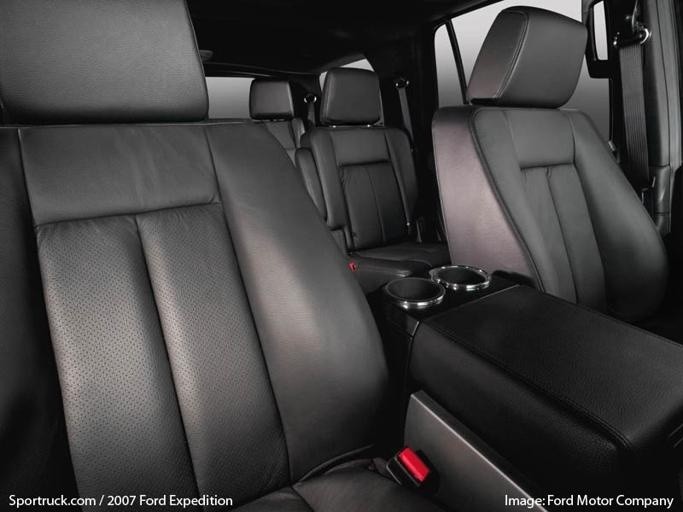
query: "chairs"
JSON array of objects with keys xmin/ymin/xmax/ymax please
[{"xmin": 2, "ymin": 0, "xmax": 680, "ymax": 511}]
